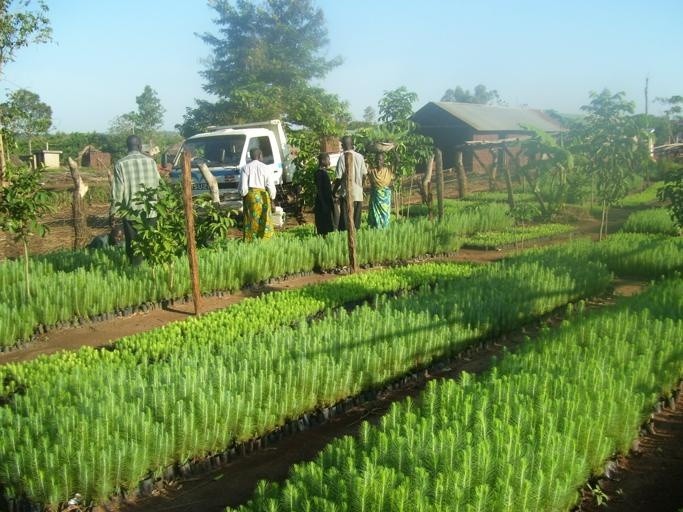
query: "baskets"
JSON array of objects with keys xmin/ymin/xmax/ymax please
[{"xmin": 368, "ymin": 141, "xmax": 394, "ymax": 154}]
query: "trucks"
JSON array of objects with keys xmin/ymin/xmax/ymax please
[{"xmin": 162, "ymin": 119, "xmax": 343, "ymax": 212}]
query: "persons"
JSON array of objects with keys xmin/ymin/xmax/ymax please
[
  {"xmin": 365, "ymin": 152, "xmax": 394, "ymax": 229},
  {"xmin": 108, "ymin": 135, "xmax": 160, "ymax": 267},
  {"xmin": 85, "ymin": 228, "xmax": 123, "ymax": 248},
  {"xmin": 238, "ymin": 148, "xmax": 277, "ymax": 241},
  {"xmin": 332, "ymin": 135, "xmax": 368, "ymax": 232},
  {"xmin": 314, "ymin": 153, "xmax": 340, "ymax": 234}
]
